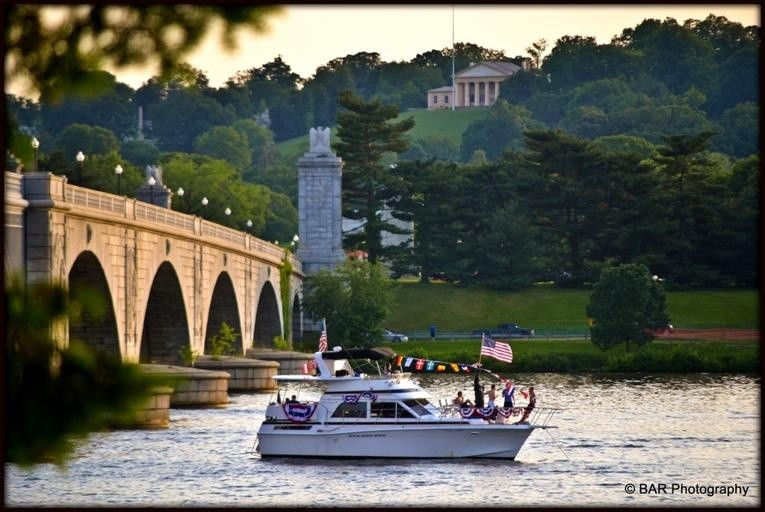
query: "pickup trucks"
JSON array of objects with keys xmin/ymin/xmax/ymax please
[{"xmin": 472, "ymin": 322, "xmax": 536, "ymax": 340}]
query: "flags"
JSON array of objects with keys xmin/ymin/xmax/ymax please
[
  {"xmin": 318, "ymin": 319, "xmax": 327, "ymax": 353},
  {"xmin": 481, "ymin": 333, "xmax": 513, "ymax": 363}
]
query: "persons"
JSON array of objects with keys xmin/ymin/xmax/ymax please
[
  {"xmin": 286, "ymin": 398, "xmax": 290, "ymax": 404},
  {"xmin": 515, "ymin": 387, "xmax": 536, "ymax": 425},
  {"xmin": 430, "ymin": 324, "xmax": 436, "ymax": 341},
  {"xmin": 454, "ymin": 391, "xmax": 476, "ymax": 407},
  {"xmin": 484, "ymin": 385, "xmax": 499, "ymax": 407},
  {"xmin": 288, "ymin": 395, "xmax": 299, "ymax": 403},
  {"xmin": 502, "ymin": 382, "xmax": 515, "ymax": 407}
]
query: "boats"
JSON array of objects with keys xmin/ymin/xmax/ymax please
[{"xmin": 257, "ymin": 347, "xmax": 561, "ymax": 462}]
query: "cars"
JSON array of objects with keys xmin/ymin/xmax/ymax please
[
  {"xmin": 644, "ymin": 324, "xmax": 675, "ymax": 335},
  {"xmin": 376, "ymin": 329, "xmax": 409, "ymax": 344}
]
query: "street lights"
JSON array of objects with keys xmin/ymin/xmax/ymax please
[
  {"xmin": 31, "ymin": 135, "xmax": 41, "ymax": 171},
  {"xmin": 247, "ymin": 219, "xmax": 253, "ymax": 233},
  {"xmin": 201, "ymin": 196, "xmax": 209, "ymax": 220},
  {"xmin": 76, "ymin": 149, "xmax": 86, "ymax": 186},
  {"xmin": 148, "ymin": 176, "xmax": 156, "ymax": 204},
  {"xmin": 268, "ymin": 234, "xmax": 300, "ymax": 253},
  {"xmin": 225, "ymin": 206, "xmax": 232, "ymax": 227},
  {"xmin": 115, "ymin": 163, "xmax": 124, "ymax": 195},
  {"xmin": 177, "ymin": 186, "xmax": 186, "ymax": 212}
]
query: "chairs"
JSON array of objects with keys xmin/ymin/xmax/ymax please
[{"xmin": 439, "ymin": 399, "xmax": 459, "ymax": 417}]
